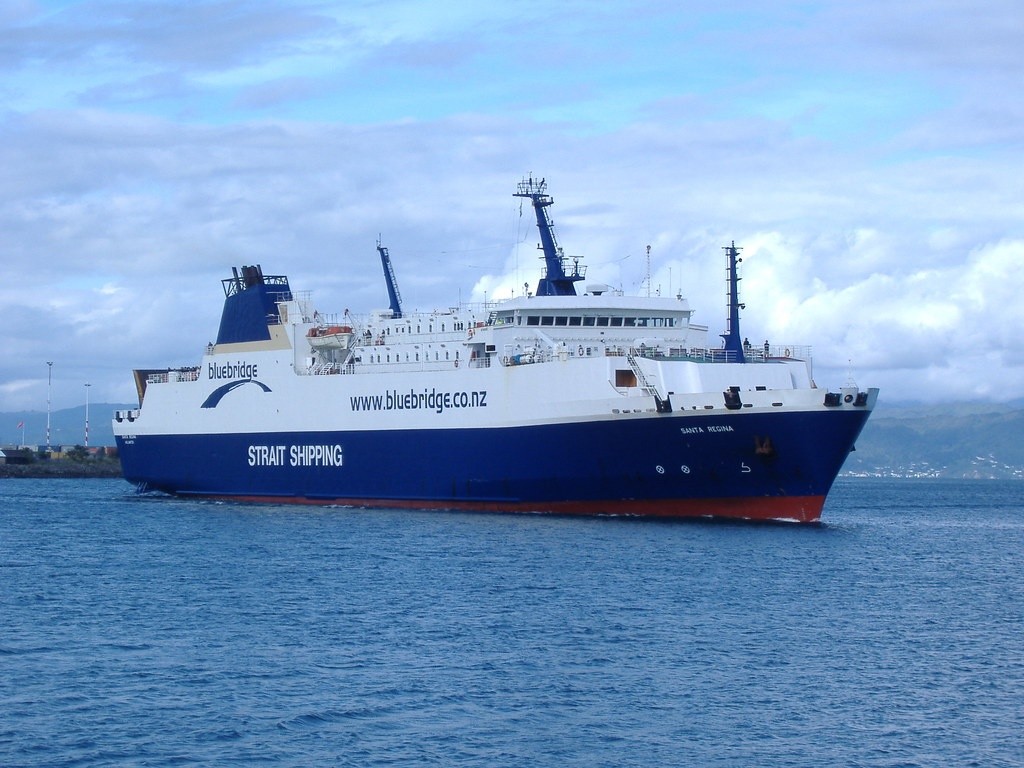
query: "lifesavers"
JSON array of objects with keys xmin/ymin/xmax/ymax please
[
  {"xmin": 785, "ymin": 349, "xmax": 791, "ymax": 356},
  {"xmin": 455, "ymin": 360, "xmax": 458, "ymax": 367},
  {"xmin": 578, "ymin": 348, "xmax": 584, "ymax": 356}
]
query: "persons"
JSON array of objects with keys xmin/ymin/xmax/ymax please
[
  {"xmin": 764, "ymin": 340, "xmax": 770, "ymax": 357},
  {"xmin": 743, "ymin": 338, "xmax": 749, "ymax": 353},
  {"xmin": 208, "ymin": 342, "xmax": 214, "ymax": 351},
  {"xmin": 496, "ymin": 317, "xmax": 502, "ymax": 325},
  {"xmin": 363, "ymin": 330, "xmax": 373, "ymax": 346},
  {"xmin": 167, "ymin": 366, "xmax": 200, "ymax": 382},
  {"xmin": 640, "ymin": 343, "xmax": 646, "ymax": 357},
  {"xmin": 488, "ymin": 317, "xmax": 492, "ymax": 325}
]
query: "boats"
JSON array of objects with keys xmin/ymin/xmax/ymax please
[
  {"xmin": 305, "ymin": 326, "xmax": 354, "ymax": 351},
  {"xmin": 109, "ymin": 167, "xmax": 881, "ymax": 524}
]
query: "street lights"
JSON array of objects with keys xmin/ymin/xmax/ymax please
[
  {"xmin": 46, "ymin": 361, "xmax": 53, "ymax": 447},
  {"xmin": 84, "ymin": 382, "xmax": 92, "ymax": 446}
]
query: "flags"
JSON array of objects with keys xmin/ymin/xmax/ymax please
[{"xmin": 16, "ymin": 421, "xmax": 25, "ymax": 429}]
